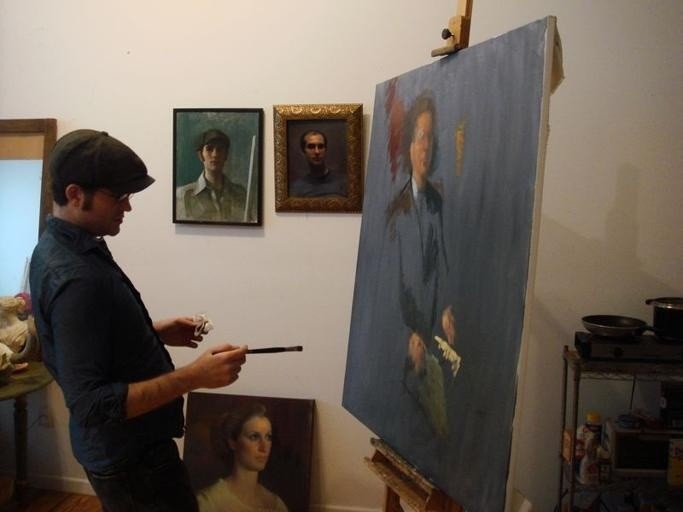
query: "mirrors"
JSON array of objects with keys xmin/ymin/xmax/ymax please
[{"xmin": 0, "ymin": 118, "xmax": 57, "ymax": 296}]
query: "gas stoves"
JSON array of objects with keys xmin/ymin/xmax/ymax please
[{"xmin": 573, "ymin": 331, "xmax": 682, "ymax": 369}]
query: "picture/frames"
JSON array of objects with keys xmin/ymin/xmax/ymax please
[
  {"xmin": 273, "ymin": 103, "xmax": 365, "ymax": 213},
  {"xmin": 172, "ymin": 107, "xmax": 264, "ymax": 227}
]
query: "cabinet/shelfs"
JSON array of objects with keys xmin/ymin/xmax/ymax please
[{"xmin": 558, "ymin": 344, "xmax": 682, "ymax": 511}]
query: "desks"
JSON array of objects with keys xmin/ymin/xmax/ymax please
[{"xmin": 0, "ymin": 361, "xmax": 54, "ymax": 510}]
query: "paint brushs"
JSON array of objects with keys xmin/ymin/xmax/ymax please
[{"xmin": 211, "ymin": 346, "xmax": 303, "ymax": 355}]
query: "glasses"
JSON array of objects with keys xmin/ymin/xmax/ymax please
[{"xmin": 104, "ymin": 191, "xmax": 134, "ymax": 199}]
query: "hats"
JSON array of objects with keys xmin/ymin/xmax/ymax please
[{"xmin": 49, "ymin": 129, "xmax": 156, "ymax": 193}]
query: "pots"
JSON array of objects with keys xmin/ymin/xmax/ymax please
[
  {"xmin": 645, "ymin": 296, "xmax": 683, "ymax": 348},
  {"xmin": 582, "ymin": 315, "xmax": 654, "ymax": 342}
]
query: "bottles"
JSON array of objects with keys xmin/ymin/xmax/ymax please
[
  {"xmin": 659, "ymin": 380, "xmax": 682, "ymax": 431},
  {"xmin": 667, "ymin": 438, "xmax": 683, "ymax": 487},
  {"xmin": 580, "ymin": 410, "xmax": 615, "ymax": 486},
  {"xmin": 618, "ymin": 488, "xmax": 658, "ymax": 512}
]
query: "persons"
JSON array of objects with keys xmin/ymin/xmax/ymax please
[
  {"xmin": 193, "ymin": 404, "xmax": 288, "ymax": 511},
  {"xmin": 383, "ymin": 97, "xmax": 456, "ymax": 454},
  {"xmin": 289, "ymin": 129, "xmax": 351, "ymax": 199},
  {"xmin": 28, "ymin": 129, "xmax": 247, "ymax": 512},
  {"xmin": 176, "ymin": 129, "xmax": 245, "ymax": 222}
]
